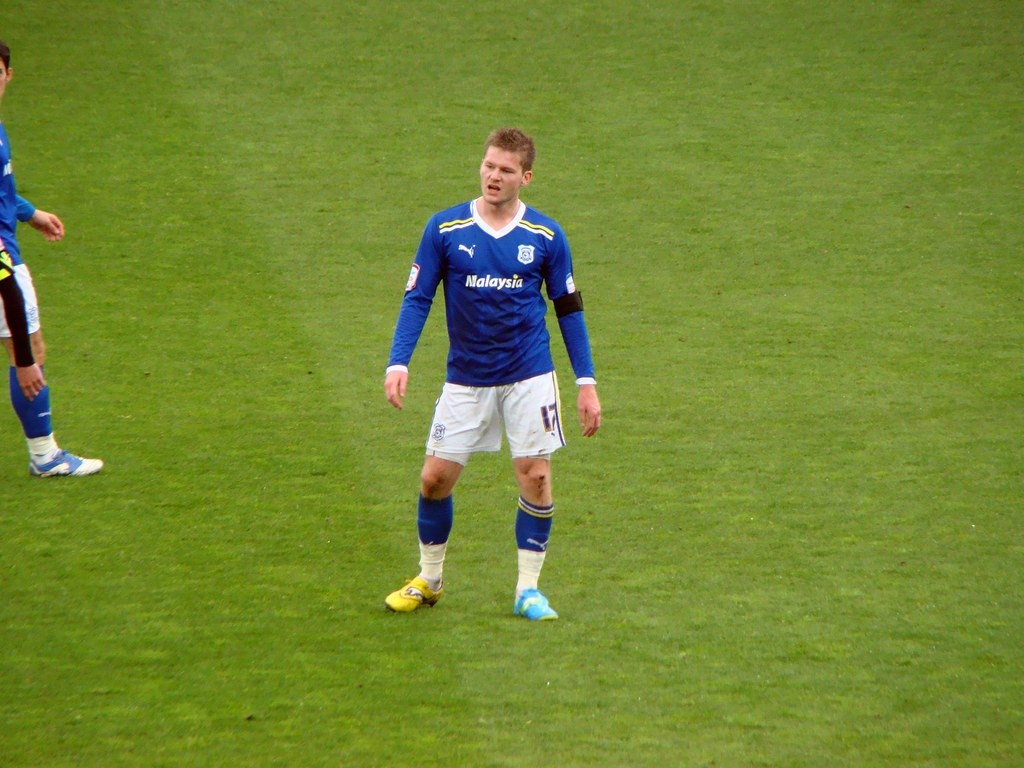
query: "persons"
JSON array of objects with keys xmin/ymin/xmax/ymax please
[
  {"xmin": 384, "ymin": 127, "xmax": 601, "ymax": 622},
  {"xmin": 0, "ymin": 40, "xmax": 104, "ymax": 479}
]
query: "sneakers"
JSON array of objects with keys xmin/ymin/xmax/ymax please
[
  {"xmin": 513, "ymin": 588, "xmax": 558, "ymax": 620},
  {"xmin": 28, "ymin": 448, "xmax": 104, "ymax": 478},
  {"xmin": 385, "ymin": 575, "xmax": 444, "ymax": 612}
]
